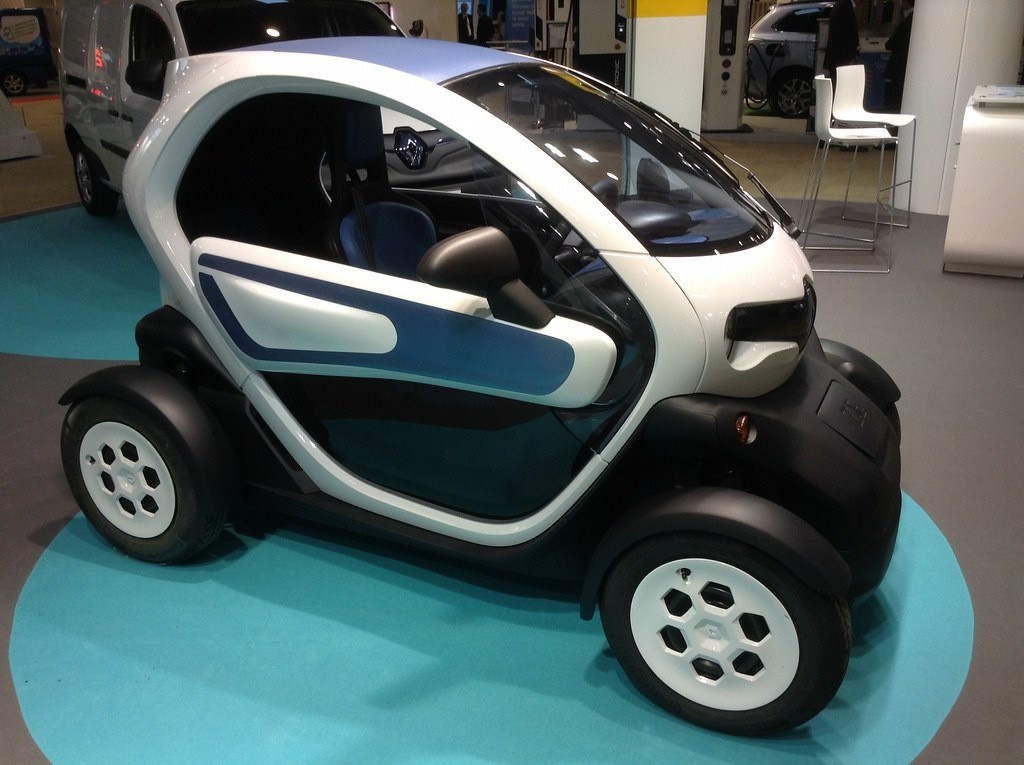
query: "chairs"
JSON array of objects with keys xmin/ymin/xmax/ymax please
[
  {"xmin": 419, "ymin": 226, "xmax": 555, "ymax": 326},
  {"xmin": 798, "ymin": 72, "xmax": 901, "ymax": 273},
  {"xmin": 312, "ymin": 137, "xmax": 439, "ymax": 280},
  {"xmin": 802, "ymin": 63, "xmax": 918, "ymax": 243}
]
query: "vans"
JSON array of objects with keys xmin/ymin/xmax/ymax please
[{"xmin": 58, "ymin": 0, "xmax": 514, "ymax": 233}]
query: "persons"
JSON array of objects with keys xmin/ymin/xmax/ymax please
[
  {"xmin": 491, "ymin": 23, "xmax": 504, "ymax": 47},
  {"xmin": 873, "ymin": 0, "xmax": 914, "ymax": 151},
  {"xmin": 819, "ymin": 0, "xmax": 868, "ymax": 153},
  {"xmin": 458, "ymin": 2, "xmax": 475, "ymax": 44},
  {"xmin": 476, "ymin": 4, "xmax": 495, "ymax": 48},
  {"xmin": 493, "ymin": 12, "xmax": 505, "ymax": 35}
]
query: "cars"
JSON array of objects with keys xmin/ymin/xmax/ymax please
[
  {"xmin": 59, "ymin": 35, "xmax": 901, "ymax": 738},
  {"xmin": 748, "ymin": 2, "xmax": 835, "ymax": 118}
]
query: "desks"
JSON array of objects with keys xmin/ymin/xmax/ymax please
[{"xmin": 941, "ymin": 95, "xmax": 1024, "ymax": 278}]
